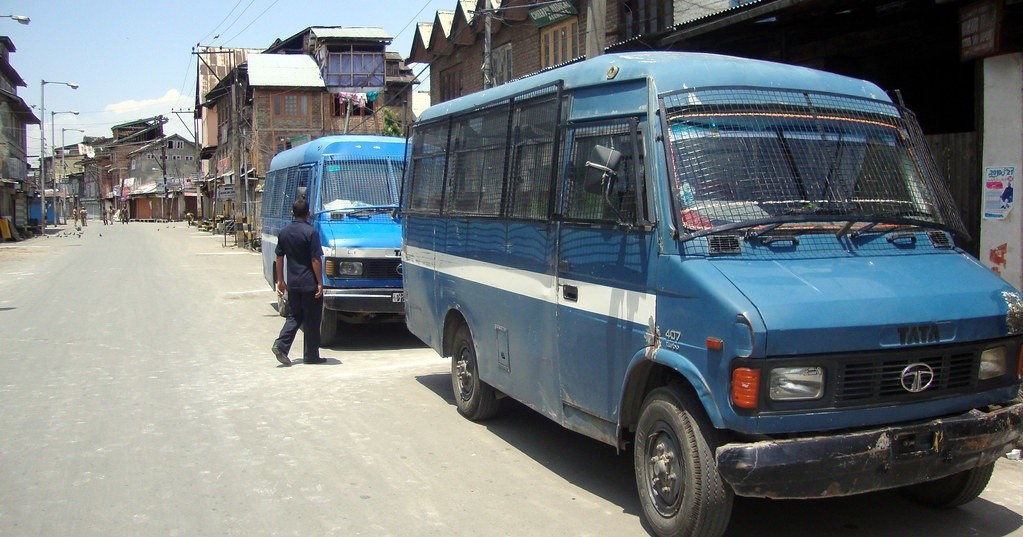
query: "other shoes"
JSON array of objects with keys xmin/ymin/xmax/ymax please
[
  {"xmin": 271, "ymin": 343, "xmax": 292, "ymax": 365},
  {"xmin": 304, "ymin": 358, "xmax": 327, "ymax": 365}
]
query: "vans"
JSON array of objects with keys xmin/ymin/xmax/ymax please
[
  {"xmin": 398, "ymin": 50, "xmax": 1023, "ymax": 536},
  {"xmin": 259, "ymin": 134, "xmax": 444, "ymax": 347}
]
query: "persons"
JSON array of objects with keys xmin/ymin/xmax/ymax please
[
  {"xmin": 73, "ymin": 206, "xmax": 78, "ymax": 226},
  {"xmin": 185, "ymin": 211, "xmax": 193, "ymax": 226},
  {"xmin": 1000, "ymin": 182, "xmax": 1013, "ymax": 209},
  {"xmin": 103, "ymin": 207, "xmax": 108, "ymax": 226},
  {"xmin": 272, "ymin": 199, "xmax": 327, "ymax": 364},
  {"xmin": 741, "ymin": 141, "xmax": 826, "ymax": 202},
  {"xmin": 108, "ymin": 205, "xmax": 116, "ymax": 225},
  {"xmin": 121, "ymin": 204, "xmax": 128, "ymax": 224},
  {"xmin": 80, "ymin": 207, "xmax": 88, "ymax": 226}
]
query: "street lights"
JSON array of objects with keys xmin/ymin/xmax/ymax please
[
  {"xmin": 51, "ymin": 112, "xmax": 80, "ymax": 226},
  {"xmin": 61, "ymin": 129, "xmax": 85, "ymax": 224},
  {"xmin": 153, "ymin": 168, "xmax": 170, "ymax": 221},
  {"xmin": 39, "ymin": 81, "xmax": 79, "ymax": 235}
]
